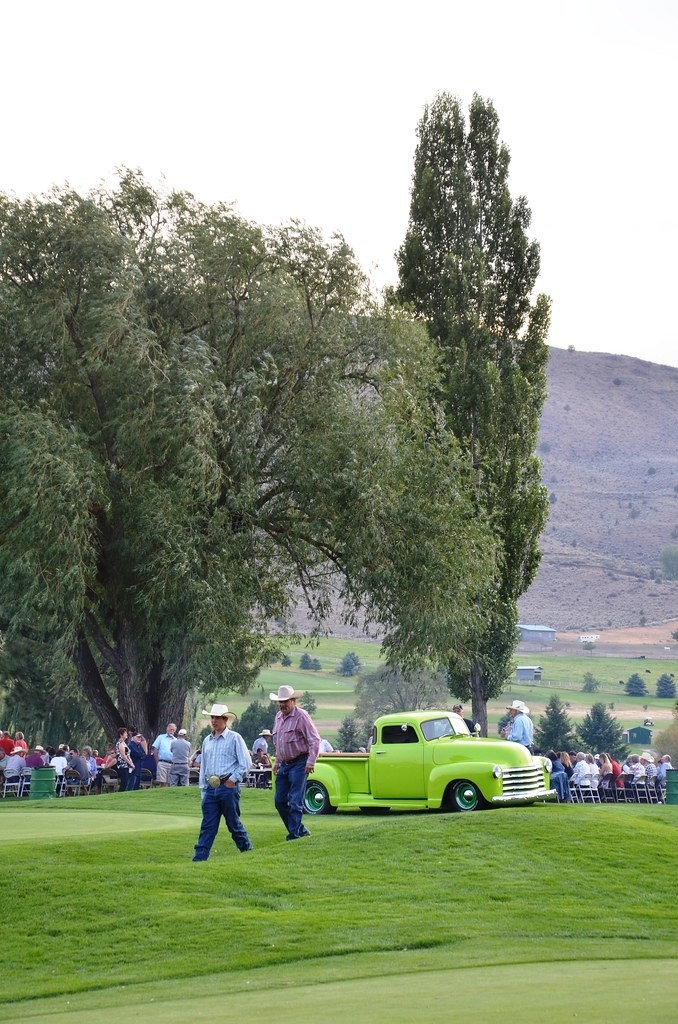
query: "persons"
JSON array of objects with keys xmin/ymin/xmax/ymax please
[
  {"xmin": 269, "ymin": 685, "xmax": 320, "ymax": 841},
  {"xmin": 506, "ymin": 701, "xmax": 534, "ymax": 754},
  {"xmin": 318, "ymin": 731, "xmax": 334, "ymax": 753},
  {"xmin": 169, "ymin": 729, "xmax": 191, "ymax": 787},
  {"xmin": 547, "ymin": 750, "xmax": 564, "ymax": 773},
  {"xmin": 560, "ymin": 752, "xmax": 574, "ymax": 780},
  {"xmin": 115, "ymin": 727, "xmax": 156, "ymax": 791},
  {"xmin": 569, "ymin": 752, "xmax": 576, "ymax": 767},
  {"xmin": 253, "ymin": 729, "xmax": 272, "ymax": 754},
  {"xmin": 498, "ymin": 702, "xmax": 525, "ymax": 739},
  {"xmin": 153, "ymin": 723, "xmax": 177, "ymax": 787},
  {"xmin": 0, "ymin": 731, "xmax": 117, "ymax": 797},
  {"xmin": 570, "ymin": 752, "xmax": 590, "ymax": 802},
  {"xmin": 585, "ymin": 752, "xmax": 674, "ymax": 804},
  {"xmin": 191, "ymin": 751, "xmax": 201, "ymax": 767},
  {"xmin": 446, "ymin": 704, "xmax": 480, "ymax": 737},
  {"xmin": 192, "ymin": 704, "xmax": 252, "ymax": 862},
  {"xmin": 253, "ymin": 748, "xmax": 270, "ymax": 788}
]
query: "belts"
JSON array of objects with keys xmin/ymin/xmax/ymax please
[
  {"xmin": 526, "ymin": 745, "xmax": 532, "ymax": 747},
  {"xmin": 158, "ymin": 760, "xmax": 171, "ymax": 763},
  {"xmin": 282, "ymin": 752, "xmax": 307, "ymax": 765},
  {"xmin": 172, "ymin": 762, "xmax": 187, "ymax": 764}
]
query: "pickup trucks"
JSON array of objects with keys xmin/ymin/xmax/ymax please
[{"xmin": 274, "ymin": 710, "xmax": 558, "ymax": 814}]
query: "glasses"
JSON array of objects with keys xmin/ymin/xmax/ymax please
[{"xmin": 70, "ymin": 753, "xmax": 73, "ymax": 755}]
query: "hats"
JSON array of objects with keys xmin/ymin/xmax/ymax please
[
  {"xmin": 59, "ymin": 744, "xmax": 64, "ymax": 750},
  {"xmin": 259, "ymin": 729, "xmax": 273, "ymax": 736},
  {"xmin": 594, "ymin": 754, "xmax": 601, "ymax": 759},
  {"xmin": 132, "ymin": 732, "xmax": 142, "ymax": 737},
  {"xmin": 178, "ymin": 729, "xmax": 187, "ymax": 735},
  {"xmin": 202, "ymin": 704, "xmax": 237, "ymax": 720},
  {"xmin": 31, "ymin": 745, "xmax": 46, "ymax": 755},
  {"xmin": 269, "ymin": 685, "xmax": 303, "ymax": 702},
  {"xmin": 506, "ymin": 700, "xmax": 530, "ymax": 714},
  {"xmin": 640, "ymin": 752, "xmax": 655, "ymax": 762},
  {"xmin": 10, "ymin": 747, "xmax": 25, "ymax": 753},
  {"xmin": 452, "ymin": 704, "xmax": 462, "ymax": 709}
]
